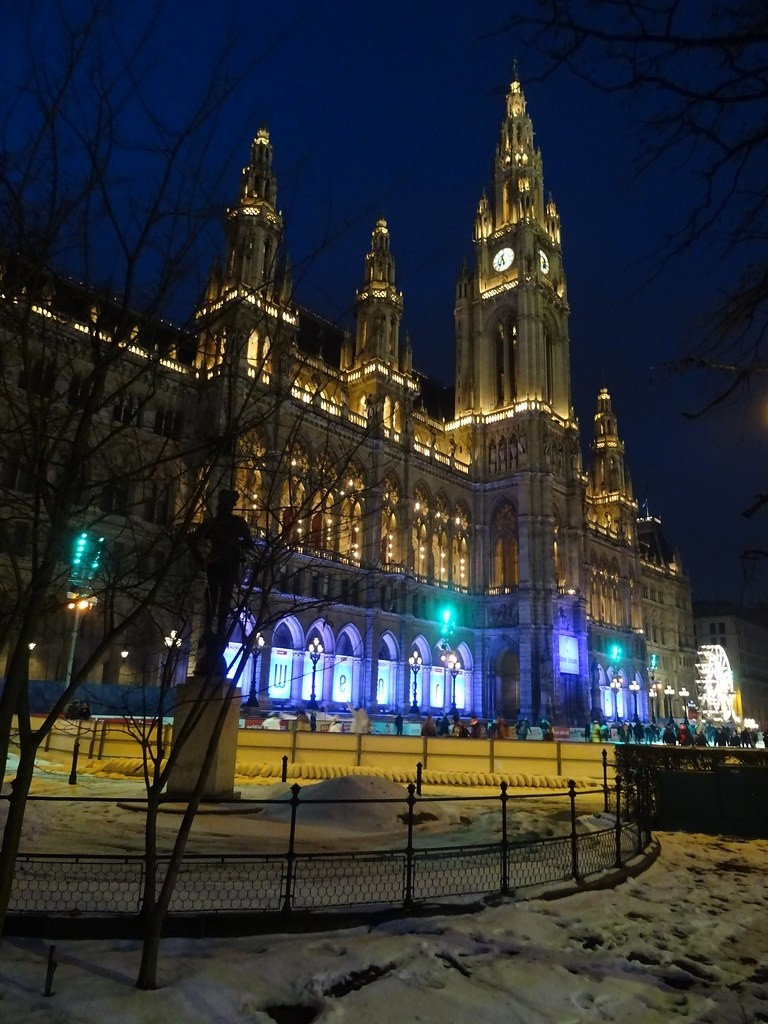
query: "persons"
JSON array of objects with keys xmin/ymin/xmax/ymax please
[
  {"xmin": 64, "ymin": 700, "xmax": 91, "ymax": 720},
  {"xmin": 196, "ymin": 492, "xmax": 253, "ymax": 650},
  {"xmin": 309, "ymin": 711, "xmax": 318, "ymax": 731},
  {"xmin": 421, "ymin": 710, "xmax": 553, "ymax": 741},
  {"xmin": 393, "ymin": 712, "xmax": 403, "ymax": 733},
  {"xmin": 584, "ymin": 720, "xmax": 768, "ymax": 748}
]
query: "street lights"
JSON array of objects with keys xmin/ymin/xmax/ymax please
[
  {"xmin": 629, "ymin": 680, "xmax": 642, "ymax": 723},
  {"xmin": 308, "ymin": 636, "xmax": 324, "ymax": 710},
  {"xmin": 664, "ymin": 684, "xmax": 676, "ymax": 725},
  {"xmin": 439, "ymin": 606, "xmax": 456, "ymax": 719},
  {"xmin": 65, "ymin": 590, "xmax": 98, "ymax": 696},
  {"xmin": 241, "ymin": 632, "xmax": 266, "ymax": 709},
  {"xmin": 648, "ymin": 683, "xmax": 662, "ymax": 724},
  {"xmin": 647, "ymin": 652, "xmax": 659, "ymax": 681},
  {"xmin": 678, "ymin": 687, "xmax": 690, "ymax": 727},
  {"xmin": 447, "ymin": 654, "xmax": 462, "ymax": 720},
  {"xmin": 610, "ymin": 675, "xmax": 623, "ymax": 729},
  {"xmin": 408, "ymin": 650, "xmax": 424, "ymax": 714}
]
